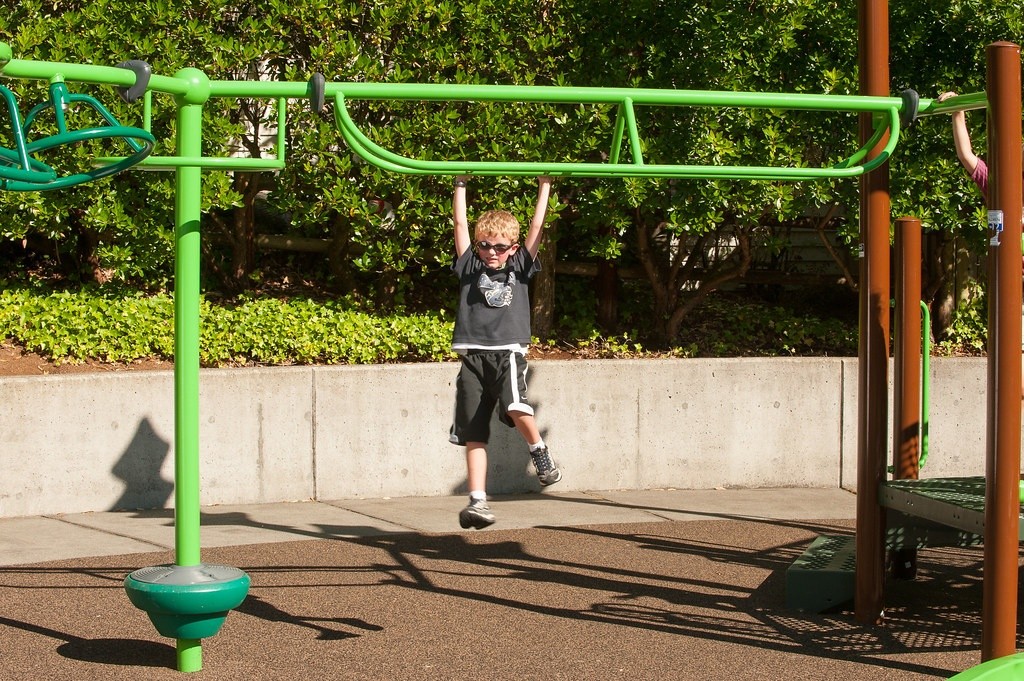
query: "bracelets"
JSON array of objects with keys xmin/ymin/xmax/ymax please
[{"xmin": 452, "ymin": 181, "xmax": 467, "ymax": 188}]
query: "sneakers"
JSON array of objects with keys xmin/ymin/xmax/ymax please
[
  {"xmin": 528, "ymin": 444, "xmax": 562, "ymax": 487},
  {"xmin": 459, "ymin": 495, "xmax": 496, "ymax": 530}
]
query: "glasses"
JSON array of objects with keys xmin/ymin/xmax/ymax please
[{"xmin": 474, "ymin": 240, "xmax": 520, "ymax": 254}]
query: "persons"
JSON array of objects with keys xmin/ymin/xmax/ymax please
[
  {"xmin": 449, "ymin": 175, "xmax": 562, "ymax": 530},
  {"xmin": 940, "ymin": 91, "xmax": 989, "ymax": 202}
]
